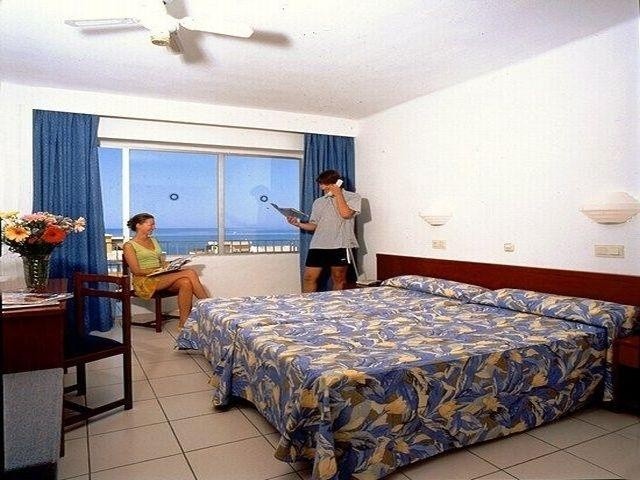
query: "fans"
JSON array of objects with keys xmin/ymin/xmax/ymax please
[{"xmin": 64, "ymin": 0, "xmax": 254, "ymax": 54}]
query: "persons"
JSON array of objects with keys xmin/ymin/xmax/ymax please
[
  {"xmin": 122, "ymin": 213, "xmax": 208, "ymax": 330},
  {"xmin": 287, "ymin": 169, "xmax": 362, "ymax": 292}
]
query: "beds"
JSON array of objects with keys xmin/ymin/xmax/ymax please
[{"xmin": 194, "ymin": 252, "xmax": 640, "ymax": 480}]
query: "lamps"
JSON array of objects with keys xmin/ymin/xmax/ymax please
[
  {"xmin": 579, "ymin": 191, "xmax": 639, "ymax": 224},
  {"xmin": 418, "ymin": 208, "xmax": 452, "ymax": 226}
]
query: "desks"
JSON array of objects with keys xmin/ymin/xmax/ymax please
[{"xmin": 1, "ymin": 278, "xmax": 69, "ymax": 457}]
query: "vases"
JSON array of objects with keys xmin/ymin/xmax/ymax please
[{"xmin": 22, "ymin": 254, "xmax": 51, "ymax": 299}]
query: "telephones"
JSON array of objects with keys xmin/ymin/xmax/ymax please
[{"xmin": 325, "ymin": 179, "xmax": 344, "ymax": 198}]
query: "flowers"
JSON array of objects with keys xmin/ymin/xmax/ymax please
[{"xmin": 0, "ymin": 208, "xmax": 87, "ymax": 285}]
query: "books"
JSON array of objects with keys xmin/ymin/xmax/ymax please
[
  {"xmin": 145, "ymin": 256, "xmax": 196, "ymax": 277},
  {"xmin": 270, "ymin": 203, "xmax": 309, "ymax": 222}
]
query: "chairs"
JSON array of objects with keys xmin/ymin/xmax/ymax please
[
  {"xmin": 121, "ymin": 253, "xmax": 180, "ymax": 332},
  {"xmin": 65, "ymin": 272, "xmax": 133, "ymax": 425}
]
